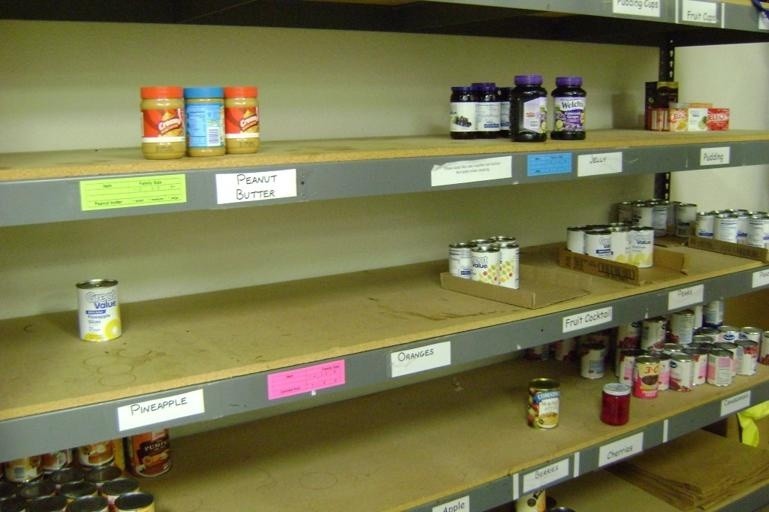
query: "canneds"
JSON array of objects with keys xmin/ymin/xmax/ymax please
[
  {"xmin": 76, "ymin": 280, "xmax": 123, "ymax": 342},
  {"xmin": 567, "ymin": 199, "xmax": 769, "ymax": 270},
  {"xmin": 450, "ymin": 82, "xmax": 512, "ymax": 138},
  {"xmin": 512, "ymin": 490, "xmax": 577, "ymax": 512},
  {"xmin": 141, "ymin": 85, "xmax": 260, "ymax": 160},
  {"xmin": 448, "ymin": 236, "xmax": 520, "ymax": 290},
  {"xmin": 527, "ymin": 298, "xmax": 768, "ymax": 430},
  {"xmin": 0, "ymin": 427, "xmax": 172, "ymax": 512}
]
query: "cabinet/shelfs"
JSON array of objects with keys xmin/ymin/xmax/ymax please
[{"xmin": 0, "ymin": 1, "xmax": 769, "ymax": 511}]
